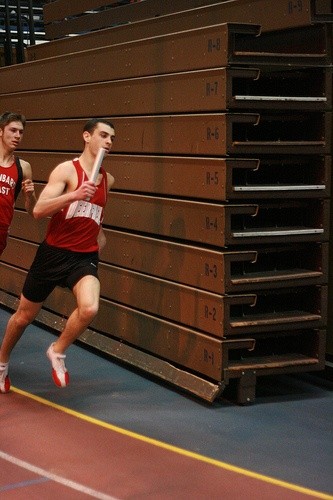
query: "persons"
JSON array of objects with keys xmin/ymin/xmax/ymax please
[
  {"xmin": 0, "ymin": 119, "xmax": 117, "ymax": 394},
  {"xmin": 1, "ymin": 110, "xmax": 38, "ymax": 259}
]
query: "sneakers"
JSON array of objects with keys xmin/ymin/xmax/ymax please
[
  {"xmin": 45, "ymin": 341, "xmax": 69, "ymax": 388},
  {"xmin": 0, "ymin": 362, "xmax": 10, "ymax": 394}
]
